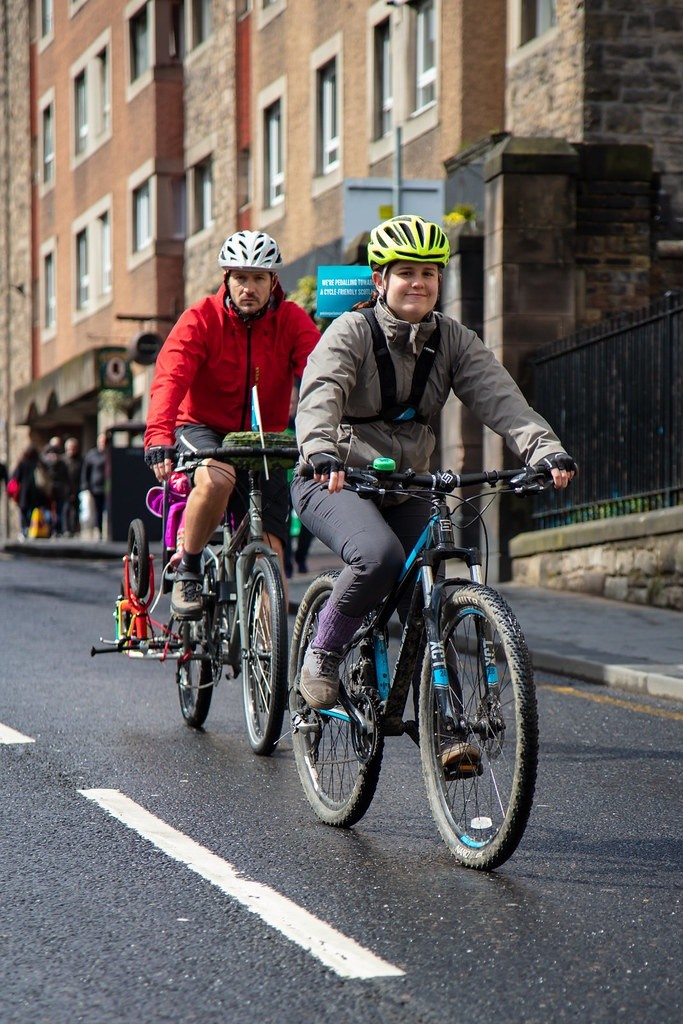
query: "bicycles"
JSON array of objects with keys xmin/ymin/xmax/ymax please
[
  {"xmin": 152, "ymin": 446, "xmax": 305, "ymax": 758},
  {"xmin": 286, "ymin": 457, "xmax": 579, "ymax": 871},
  {"xmin": 90, "ymin": 519, "xmax": 197, "ymax": 659}
]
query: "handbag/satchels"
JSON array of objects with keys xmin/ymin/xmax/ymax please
[
  {"xmin": 80, "ymin": 490, "xmax": 96, "ymax": 526},
  {"xmin": 29, "ymin": 508, "xmax": 49, "ymax": 537}
]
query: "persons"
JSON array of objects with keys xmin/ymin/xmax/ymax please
[
  {"xmin": 145, "ymin": 229, "xmax": 322, "ymax": 713},
  {"xmin": 0, "ymin": 432, "xmax": 108, "ymax": 543},
  {"xmin": 289, "ymin": 215, "xmax": 579, "ymax": 780}
]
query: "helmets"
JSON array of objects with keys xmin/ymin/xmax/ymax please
[
  {"xmin": 367, "ymin": 215, "xmax": 450, "ymax": 270},
  {"xmin": 218, "ymin": 230, "xmax": 283, "ymax": 272}
]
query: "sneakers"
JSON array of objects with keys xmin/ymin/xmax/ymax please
[
  {"xmin": 301, "ymin": 641, "xmax": 346, "ymax": 709},
  {"xmin": 440, "ymin": 741, "xmax": 481, "ymax": 766},
  {"xmin": 165, "ymin": 567, "xmax": 204, "ymax": 620}
]
